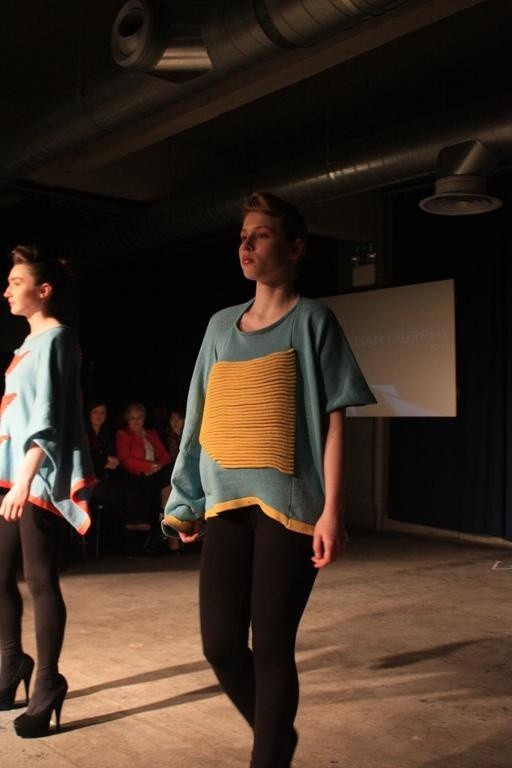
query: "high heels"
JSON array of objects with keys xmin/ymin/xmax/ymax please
[
  {"xmin": 1, "ymin": 653, "xmax": 35, "ymax": 710},
  {"xmin": 14, "ymin": 673, "xmax": 67, "ymax": 738}
]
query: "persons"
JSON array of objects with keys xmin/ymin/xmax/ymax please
[
  {"xmin": 0, "ymin": 244, "xmax": 101, "ymax": 737},
  {"xmin": 77, "ymin": 396, "xmax": 199, "ymax": 560},
  {"xmin": 163, "ymin": 185, "xmax": 377, "ymax": 768}
]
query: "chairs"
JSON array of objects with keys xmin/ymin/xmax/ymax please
[{"xmin": 69, "ymin": 504, "xmax": 105, "ymax": 560}]
